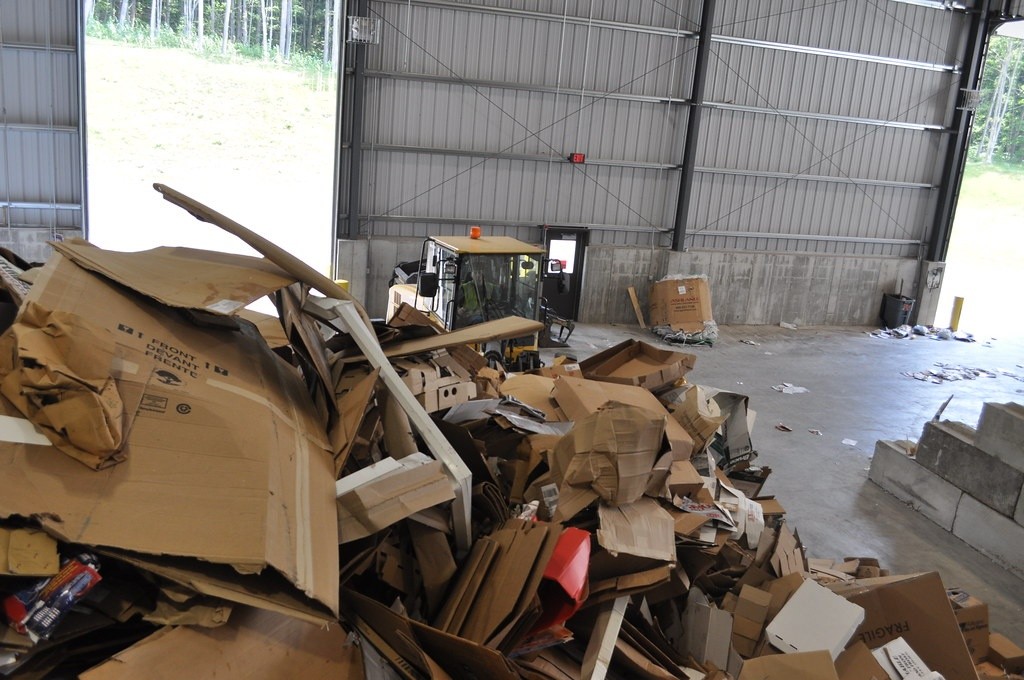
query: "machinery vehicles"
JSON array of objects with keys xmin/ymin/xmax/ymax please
[{"xmin": 386, "ymin": 225, "xmax": 572, "ymax": 370}]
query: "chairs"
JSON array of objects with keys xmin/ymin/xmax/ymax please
[{"xmin": 465, "ymin": 272, "xmax": 484, "ymax": 282}]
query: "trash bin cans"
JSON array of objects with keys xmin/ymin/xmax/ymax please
[{"xmin": 879, "ymin": 293, "xmax": 915, "ymax": 329}]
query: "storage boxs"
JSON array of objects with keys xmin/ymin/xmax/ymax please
[
  {"xmin": 829, "ymin": 571, "xmax": 1024, "ymax": 680},
  {"xmin": 581, "ymin": 337, "xmax": 695, "ymax": 388},
  {"xmin": 647, "ymin": 278, "xmax": 713, "ymax": 335}
]
